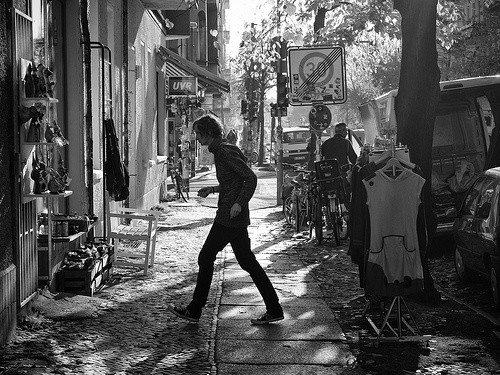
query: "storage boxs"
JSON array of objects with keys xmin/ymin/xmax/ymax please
[{"xmin": 55, "ymin": 250, "xmax": 116, "ymax": 297}]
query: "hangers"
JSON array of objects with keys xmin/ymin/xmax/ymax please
[{"xmin": 374, "ymin": 146, "xmax": 416, "ymax": 172}]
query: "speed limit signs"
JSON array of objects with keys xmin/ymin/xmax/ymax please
[{"xmin": 284, "ymin": 44, "xmax": 348, "ymax": 105}]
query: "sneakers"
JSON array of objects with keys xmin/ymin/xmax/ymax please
[
  {"xmin": 251, "ymin": 311, "xmax": 284, "ymax": 324},
  {"xmin": 168, "ymin": 304, "xmax": 200, "ymax": 322}
]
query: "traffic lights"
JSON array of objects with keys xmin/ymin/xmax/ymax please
[
  {"xmin": 249, "ymin": 101, "xmax": 258, "ymax": 123},
  {"xmin": 241, "ymin": 99, "xmax": 249, "ymax": 114}
]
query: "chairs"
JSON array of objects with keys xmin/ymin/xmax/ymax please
[
  {"xmin": 104, "ymin": 189, "xmax": 160, "ymax": 277},
  {"xmin": 315, "ymin": 159, "xmax": 349, "ymax": 208}
]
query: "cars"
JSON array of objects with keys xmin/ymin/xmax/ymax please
[
  {"xmin": 359, "ymin": 74, "xmax": 500, "ymax": 248},
  {"xmin": 448, "ymin": 167, "xmax": 500, "ymax": 296}
]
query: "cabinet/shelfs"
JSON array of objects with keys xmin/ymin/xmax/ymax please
[{"xmin": 17, "ymin": 57, "xmax": 101, "ymax": 282}]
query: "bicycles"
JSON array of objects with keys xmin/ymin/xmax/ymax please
[
  {"xmin": 281, "ymin": 161, "xmax": 353, "ymax": 244},
  {"xmin": 247, "ymin": 151, "xmax": 258, "ymax": 167},
  {"xmin": 164, "ymin": 156, "xmax": 191, "ymax": 203}
]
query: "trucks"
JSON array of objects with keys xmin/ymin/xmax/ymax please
[{"xmin": 283, "ymin": 126, "xmax": 313, "ymax": 160}]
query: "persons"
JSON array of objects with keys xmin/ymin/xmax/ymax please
[
  {"xmin": 168, "ymin": 114, "xmax": 285, "ymax": 324},
  {"xmin": 226, "ymin": 128, "xmax": 237, "ymax": 146},
  {"xmin": 313, "ymin": 122, "xmax": 360, "ymax": 227}
]
